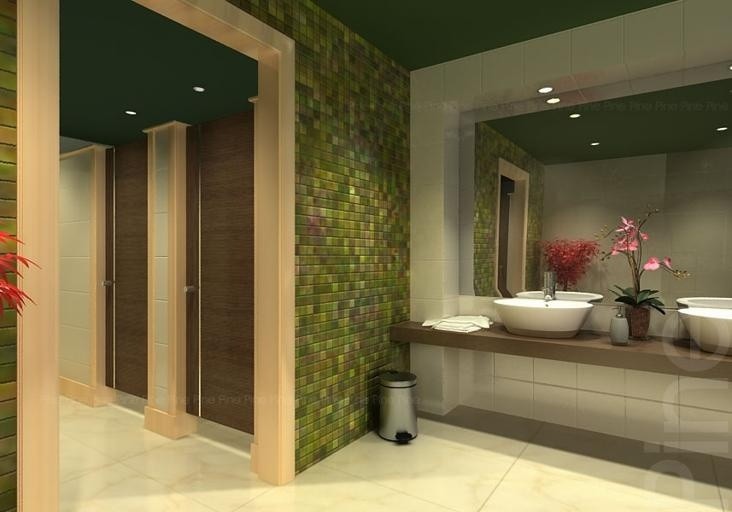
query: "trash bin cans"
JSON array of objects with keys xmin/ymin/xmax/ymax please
[{"xmin": 379, "ymin": 370, "xmax": 417, "ymax": 444}]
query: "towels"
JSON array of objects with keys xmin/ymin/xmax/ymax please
[{"xmin": 424, "ymin": 314, "xmax": 495, "ymax": 333}]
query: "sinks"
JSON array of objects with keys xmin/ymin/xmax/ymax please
[
  {"xmin": 493, "ymin": 297, "xmax": 593, "ymax": 338},
  {"xmin": 678, "ymin": 308, "xmax": 732, "ymax": 354},
  {"xmin": 516, "ymin": 290, "xmax": 604, "ymax": 302},
  {"xmin": 676, "ymin": 297, "xmax": 732, "ymax": 306}
]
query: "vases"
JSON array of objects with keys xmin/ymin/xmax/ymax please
[{"xmin": 624, "ymin": 307, "xmax": 650, "ymax": 339}]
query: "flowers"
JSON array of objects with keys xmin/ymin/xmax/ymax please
[{"xmin": 539, "ymin": 212, "xmax": 685, "ymax": 308}]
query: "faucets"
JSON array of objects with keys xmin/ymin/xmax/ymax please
[{"xmin": 543, "ymin": 271, "xmax": 555, "ymax": 300}]
